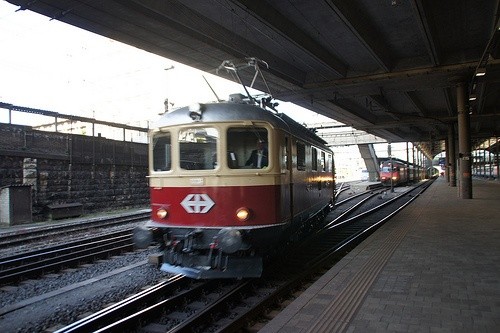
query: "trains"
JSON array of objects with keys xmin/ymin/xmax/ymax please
[
  {"xmin": 139, "ymin": 100, "xmax": 338, "ymax": 281},
  {"xmin": 381, "ymin": 161, "xmax": 428, "ymax": 186}
]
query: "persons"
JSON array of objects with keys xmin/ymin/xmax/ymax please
[{"xmin": 244, "ymin": 139, "xmax": 268, "ymax": 168}]
